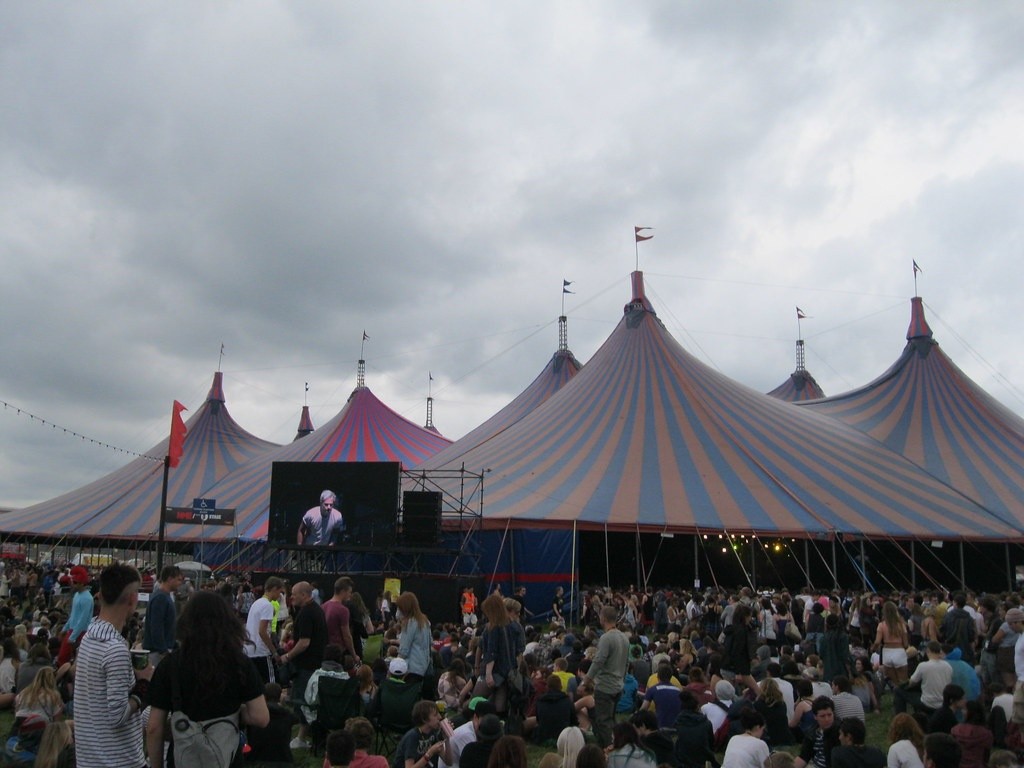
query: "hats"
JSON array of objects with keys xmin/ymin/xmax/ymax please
[
  {"xmin": 475, "ymin": 713, "xmax": 503, "ymax": 740},
  {"xmin": 1004, "ymin": 608, "xmax": 1024, "ymax": 622},
  {"xmin": 468, "ymin": 697, "xmax": 488, "ymax": 710},
  {"xmin": 389, "ymin": 658, "xmax": 407, "ymax": 675},
  {"xmin": 715, "ymin": 680, "xmax": 735, "ymax": 701},
  {"xmin": 803, "ymin": 667, "xmax": 819, "ymax": 679},
  {"xmin": 17, "ymin": 713, "xmax": 49, "ymax": 750}
]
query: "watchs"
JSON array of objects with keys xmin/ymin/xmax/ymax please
[{"xmin": 581, "ymin": 681, "xmax": 587, "ymax": 688}]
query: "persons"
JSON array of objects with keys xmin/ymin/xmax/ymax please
[
  {"xmin": 297, "ymin": 487, "xmax": 344, "ymax": 547},
  {"xmin": 0, "ymin": 559, "xmax": 1023, "ymax": 768}
]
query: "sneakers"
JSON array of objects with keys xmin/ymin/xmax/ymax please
[{"xmin": 289, "ymin": 736, "xmax": 312, "ymax": 749}]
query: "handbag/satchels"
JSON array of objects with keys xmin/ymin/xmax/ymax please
[
  {"xmin": 170, "ymin": 709, "xmax": 242, "ymax": 768},
  {"xmin": 507, "ymin": 676, "xmax": 533, "ymax": 719},
  {"xmin": 783, "ymin": 611, "xmax": 803, "ymax": 642},
  {"xmin": 507, "ymin": 667, "xmax": 523, "ymax": 694}
]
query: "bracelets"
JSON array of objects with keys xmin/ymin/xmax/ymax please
[
  {"xmin": 273, "ymin": 651, "xmax": 278, "ymax": 657},
  {"xmin": 286, "ymin": 653, "xmax": 291, "ymax": 662},
  {"xmin": 424, "ymin": 748, "xmax": 435, "ymax": 763}
]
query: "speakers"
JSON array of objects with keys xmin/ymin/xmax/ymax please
[{"xmin": 402, "ymin": 491, "xmax": 442, "ymax": 549}]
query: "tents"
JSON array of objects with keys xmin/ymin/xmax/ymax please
[{"xmin": 0, "ymin": 268, "xmax": 1024, "ymax": 635}]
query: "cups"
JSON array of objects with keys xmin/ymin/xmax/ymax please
[
  {"xmin": 131, "ymin": 651, "xmax": 149, "ymax": 670},
  {"xmin": 439, "ymin": 718, "xmax": 454, "ymax": 736}
]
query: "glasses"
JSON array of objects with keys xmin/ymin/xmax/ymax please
[{"xmin": 71, "ymin": 580, "xmax": 81, "ymax": 584}]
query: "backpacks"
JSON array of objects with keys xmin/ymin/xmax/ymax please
[{"xmin": 709, "ymin": 700, "xmax": 736, "ymax": 752}]
